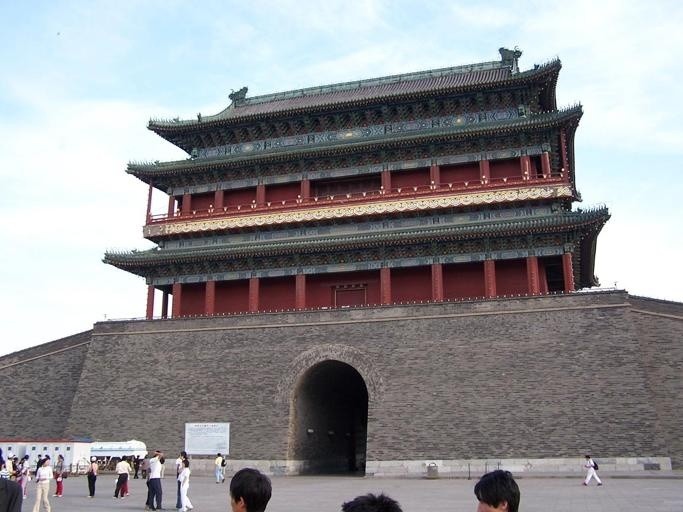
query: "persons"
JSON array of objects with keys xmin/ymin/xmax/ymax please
[
  {"xmin": 215, "ymin": 453, "xmax": 225, "ymax": 484},
  {"xmin": 473, "ymin": 469, "xmax": 521, "ymax": 512},
  {"xmin": 584, "ymin": 456, "xmax": 602, "ymax": 486},
  {"xmin": 230, "ymin": 469, "xmax": 271, "ymax": 512},
  {"xmin": 221, "ymin": 456, "xmax": 226, "ymax": 477},
  {"xmin": 341, "ymin": 494, "xmax": 402, "ymax": 512},
  {"xmin": 1, "ymin": 449, "xmax": 194, "ymax": 512}
]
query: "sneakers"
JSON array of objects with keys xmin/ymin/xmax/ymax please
[
  {"xmin": 52, "ymin": 494, "xmax": 63, "ymax": 498},
  {"xmin": 112, "ymin": 492, "xmax": 130, "ymax": 500},
  {"xmin": 216, "ymin": 482, "xmax": 220, "ymax": 484},
  {"xmin": 222, "ymin": 479, "xmax": 225, "ymax": 483},
  {"xmin": 187, "ymin": 507, "xmax": 194, "ymax": 512},
  {"xmin": 179, "ymin": 507, "xmax": 188, "ymax": 512},
  {"xmin": 23, "ymin": 495, "xmax": 27, "ymax": 500}
]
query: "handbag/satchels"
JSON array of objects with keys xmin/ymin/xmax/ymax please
[
  {"xmin": 57, "ymin": 475, "xmax": 62, "ymax": 482},
  {"xmin": 87, "ymin": 470, "xmax": 94, "ymax": 478},
  {"xmin": 61, "ymin": 471, "xmax": 68, "ymax": 478}
]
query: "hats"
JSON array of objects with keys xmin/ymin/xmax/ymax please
[
  {"xmin": 89, "ymin": 455, "xmax": 97, "ymax": 462},
  {"xmin": 7, "ymin": 452, "xmax": 15, "ymax": 459}
]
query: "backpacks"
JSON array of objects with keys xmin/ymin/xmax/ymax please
[{"xmin": 220, "ymin": 457, "xmax": 227, "ymax": 467}]
